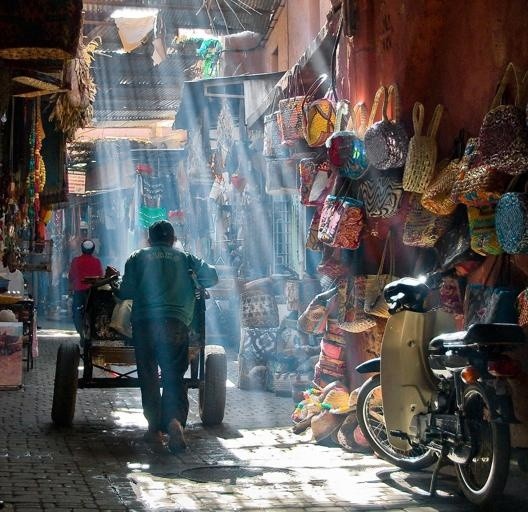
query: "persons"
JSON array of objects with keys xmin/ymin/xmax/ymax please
[
  {"xmin": 104, "ymin": 220, "xmax": 218, "ymax": 451},
  {"xmin": 0, "ymin": 252, "xmax": 25, "ymax": 317},
  {"xmin": 68, "ymin": 239, "xmax": 101, "ymax": 347}
]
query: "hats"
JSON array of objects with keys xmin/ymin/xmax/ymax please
[
  {"xmin": 81, "ymin": 240, "xmax": 95, "ymax": 250},
  {"xmin": 290, "ymin": 381, "xmax": 371, "ymax": 451},
  {"xmin": 148, "ymin": 220, "xmax": 178, "ymax": 241}
]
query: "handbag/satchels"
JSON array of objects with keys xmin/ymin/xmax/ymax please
[
  {"xmin": 476, "ymin": 63, "xmax": 528, "ymax": 176},
  {"xmin": 327, "ymin": 100, "xmax": 354, "ymax": 167},
  {"xmin": 459, "ymin": 138, "xmax": 496, "ymax": 206},
  {"xmin": 472, "ymin": 202, "xmax": 500, "ymax": 255},
  {"xmin": 464, "ymin": 255, "xmax": 512, "ymax": 324},
  {"xmin": 296, "ymin": 288, "xmax": 338, "ymax": 335},
  {"xmin": 318, "ymin": 179, "xmax": 364, "ymax": 249},
  {"xmin": 304, "ymin": 206, "xmax": 321, "ymax": 253},
  {"xmin": 423, "ymin": 138, "xmax": 466, "ymax": 214},
  {"xmin": 402, "ymin": 191, "xmax": 469, "ymax": 248},
  {"xmin": 264, "ymin": 87, "xmax": 292, "ymax": 156},
  {"xmin": 308, "ymin": 85, "xmax": 334, "ymax": 147},
  {"xmin": 364, "ymin": 178, "xmax": 404, "ymax": 218},
  {"xmin": 496, "ymin": 175, "xmax": 528, "ymax": 255},
  {"xmin": 317, "ymin": 250, "xmax": 361, "ymax": 276},
  {"xmin": 401, "ymin": 101, "xmax": 444, "ymax": 191},
  {"xmin": 466, "ymin": 255, "xmax": 504, "ymax": 285},
  {"xmin": 364, "ymin": 85, "xmax": 405, "ymax": 176},
  {"xmin": 336, "ymin": 251, "xmax": 377, "ymax": 334},
  {"xmin": 340, "ymin": 104, "xmax": 367, "ymax": 177},
  {"xmin": 363, "ymin": 231, "xmax": 401, "ymax": 319},
  {"xmin": 284, "ymin": 70, "xmax": 306, "ymax": 148},
  {"xmin": 136, "ymin": 198, "xmax": 170, "ymax": 230},
  {"xmin": 264, "ymin": 148, "xmax": 329, "ymax": 207}
]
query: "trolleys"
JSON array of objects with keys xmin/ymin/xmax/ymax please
[{"xmin": 51, "ymin": 272, "xmax": 227, "ymax": 430}]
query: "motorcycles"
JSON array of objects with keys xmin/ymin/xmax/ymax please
[{"xmin": 354, "ymin": 269, "xmax": 528, "ymax": 504}]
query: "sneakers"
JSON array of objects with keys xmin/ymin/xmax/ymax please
[
  {"xmin": 141, "ymin": 433, "xmax": 162, "ymax": 441},
  {"xmin": 169, "ymin": 419, "xmax": 188, "ymax": 457}
]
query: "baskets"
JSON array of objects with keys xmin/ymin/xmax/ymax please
[
  {"xmin": 285, "ymin": 279, "xmax": 319, "ymax": 311},
  {"xmin": 276, "ymin": 319, "xmax": 316, "ymax": 392},
  {"xmin": 237, "ymin": 276, "xmax": 277, "ymax": 392}
]
